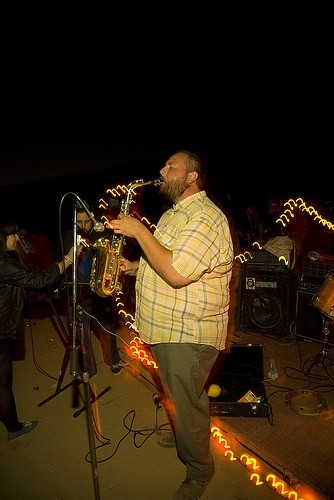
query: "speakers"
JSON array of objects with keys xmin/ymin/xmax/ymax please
[
  {"xmin": 294, "ymin": 290, "xmax": 334, "ymax": 347},
  {"xmin": 208, "ymin": 342, "xmax": 266, "ymax": 402},
  {"xmin": 235, "ymin": 269, "xmax": 290, "ymax": 338}
]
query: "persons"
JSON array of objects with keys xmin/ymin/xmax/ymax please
[
  {"xmin": 59, "ymin": 199, "xmax": 123, "ymax": 379},
  {"xmin": 238, "ymin": 205, "xmax": 276, "ymax": 235},
  {"xmin": 104, "ymin": 150, "xmax": 233, "ymax": 500},
  {"xmin": 0, "ymin": 216, "xmax": 84, "ymax": 441}
]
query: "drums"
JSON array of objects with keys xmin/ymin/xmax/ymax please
[{"xmin": 310, "ymin": 271, "xmax": 334, "ymax": 321}]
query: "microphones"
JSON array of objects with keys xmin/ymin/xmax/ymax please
[{"xmin": 77, "ymin": 196, "xmax": 105, "ymax": 233}]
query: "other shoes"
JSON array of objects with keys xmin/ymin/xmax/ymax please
[
  {"xmin": 8, "ymin": 420, "xmax": 39, "ymax": 443},
  {"xmin": 110, "ymin": 364, "xmax": 121, "ymax": 373},
  {"xmin": 173, "ymin": 469, "xmax": 215, "ymax": 500},
  {"xmin": 157, "ymin": 431, "xmax": 176, "ymax": 448}
]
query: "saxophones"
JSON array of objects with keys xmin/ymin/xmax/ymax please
[{"xmin": 86, "ymin": 178, "xmax": 163, "ymax": 298}]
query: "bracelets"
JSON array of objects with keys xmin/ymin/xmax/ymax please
[{"xmin": 64, "ymin": 256, "xmax": 73, "ymax": 264}]
